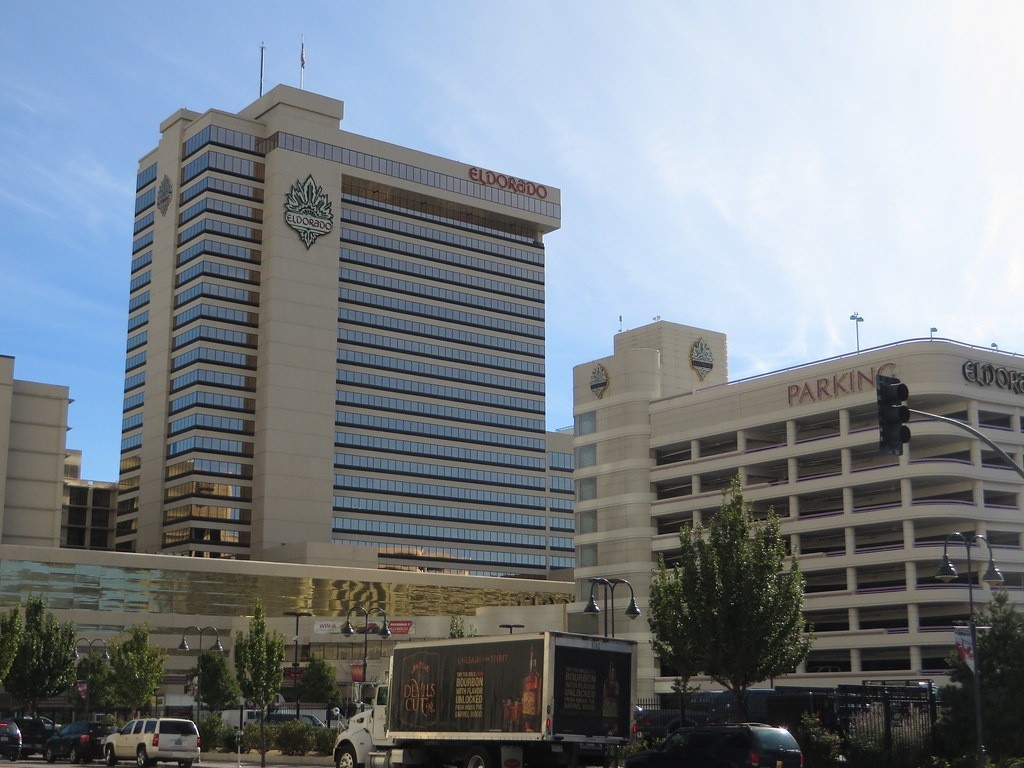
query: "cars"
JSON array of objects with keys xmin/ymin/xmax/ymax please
[
  {"xmin": 0, "ymin": 718, "xmax": 22, "ymax": 762},
  {"xmin": 12, "ymin": 716, "xmax": 62, "ymax": 759}
]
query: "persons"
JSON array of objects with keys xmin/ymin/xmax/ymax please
[{"xmin": 337, "ymin": 714, "xmax": 348, "ymax": 733}]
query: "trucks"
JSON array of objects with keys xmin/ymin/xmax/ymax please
[{"xmin": 332, "ymin": 629, "xmax": 638, "ymax": 766}]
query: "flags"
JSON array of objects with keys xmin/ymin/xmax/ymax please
[{"xmin": 301, "ymin": 45, "xmax": 306, "ymax": 69}]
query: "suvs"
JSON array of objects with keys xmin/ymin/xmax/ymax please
[
  {"xmin": 42, "ymin": 721, "xmax": 121, "ymax": 764},
  {"xmin": 263, "ymin": 713, "xmax": 325, "ymax": 729},
  {"xmin": 622, "ymin": 722, "xmax": 803, "ymax": 768},
  {"xmin": 101, "ymin": 716, "xmax": 201, "ymax": 768}
]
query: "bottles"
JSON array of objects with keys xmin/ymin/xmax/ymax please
[
  {"xmin": 601, "ymin": 662, "xmax": 620, "ymax": 733},
  {"xmin": 521, "ymin": 643, "xmax": 541, "ymax": 729}
]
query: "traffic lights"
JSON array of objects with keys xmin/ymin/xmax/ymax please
[{"xmin": 876, "ymin": 373, "xmax": 911, "ymax": 457}]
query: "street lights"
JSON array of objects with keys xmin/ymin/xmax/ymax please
[
  {"xmin": 177, "ymin": 626, "xmax": 224, "ymax": 731},
  {"xmin": 933, "ymin": 530, "xmax": 1005, "ymax": 768},
  {"xmin": 587, "ymin": 578, "xmax": 625, "ymax": 638},
  {"xmin": 583, "ymin": 577, "xmax": 642, "ymax": 638},
  {"xmin": 341, "ymin": 605, "xmax": 392, "ymax": 712},
  {"xmin": 283, "ymin": 611, "xmax": 313, "ymax": 722},
  {"xmin": 70, "ymin": 637, "xmax": 111, "ymax": 721}
]
